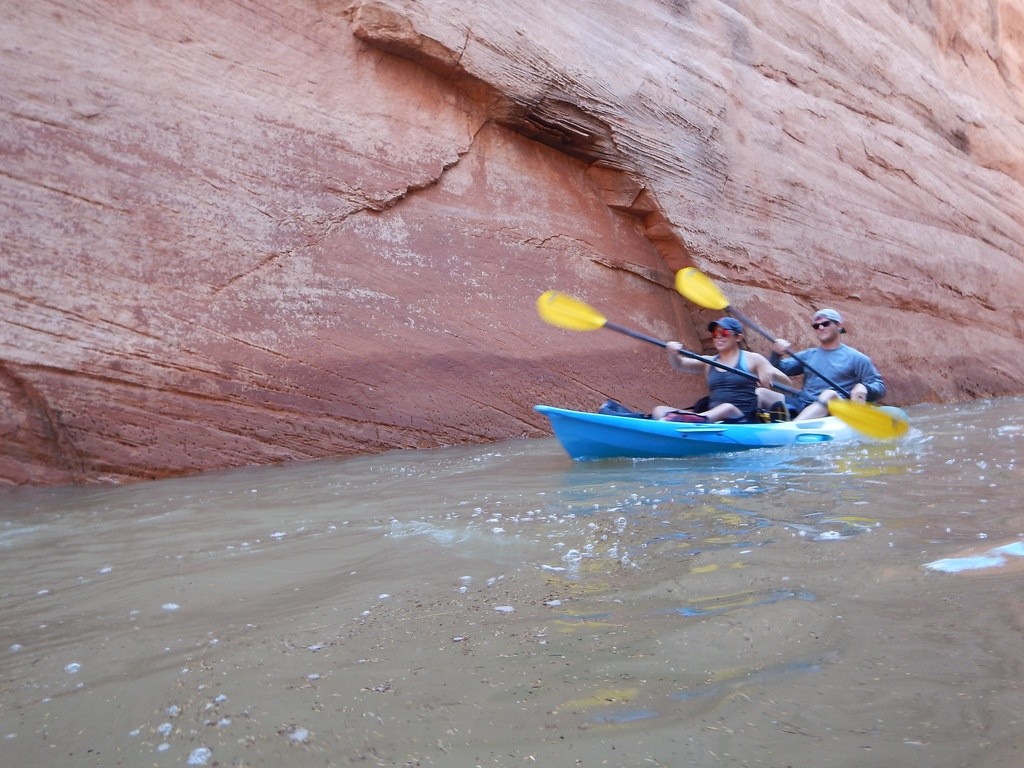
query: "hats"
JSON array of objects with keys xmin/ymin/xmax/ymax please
[
  {"xmin": 813, "ymin": 308, "xmax": 847, "ymax": 332},
  {"xmin": 708, "ymin": 317, "xmax": 744, "ymax": 334}
]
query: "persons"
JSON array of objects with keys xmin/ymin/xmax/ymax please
[
  {"xmin": 765, "ymin": 308, "xmax": 885, "ymax": 422},
  {"xmin": 652, "ymin": 316, "xmax": 793, "ymax": 425}
]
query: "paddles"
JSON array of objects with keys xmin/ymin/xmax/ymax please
[
  {"xmin": 535, "ymin": 287, "xmax": 909, "ymax": 442},
  {"xmin": 674, "ymin": 265, "xmax": 854, "ymax": 402}
]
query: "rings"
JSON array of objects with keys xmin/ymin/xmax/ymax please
[{"xmin": 859, "ymin": 396, "xmax": 863, "ymax": 398}]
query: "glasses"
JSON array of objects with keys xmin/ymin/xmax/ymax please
[
  {"xmin": 712, "ymin": 330, "xmax": 735, "ymax": 339},
  {"xmin": 811, "ymin": 321, "xmax": 835, "ymax": 330}
]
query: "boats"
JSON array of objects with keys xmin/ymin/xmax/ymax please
[{"xmin": 534, "ymin": 403, "xmax": 909, "ymax": 461}]
